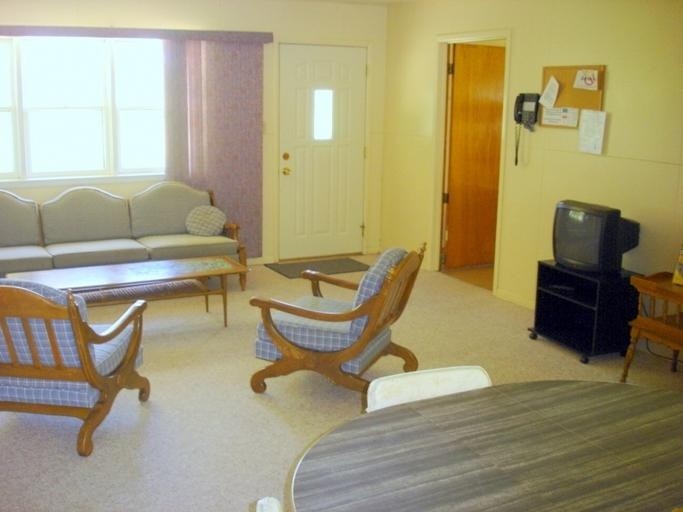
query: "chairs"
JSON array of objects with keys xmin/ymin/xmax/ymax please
[
  {"xmin": 0, "ymin": 279, "xmax": 151, "ymax": 458},
  {"xmin": 248, "ymin": 240, "xmax": 430, "ymax": 395}
]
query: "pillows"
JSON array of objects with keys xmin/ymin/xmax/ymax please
[{"xmin": 185, "ymin": 204, "xmax": 227, "ymax": 236}]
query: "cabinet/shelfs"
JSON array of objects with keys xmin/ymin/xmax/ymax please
[
  {"xmin": 618, "ymin": 271, "xmax": 683, "ymax": 384},
  {"xmin": 528, "ymin": 259, "xmax": 647, "ymax": 365}
]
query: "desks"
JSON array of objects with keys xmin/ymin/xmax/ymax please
[{"xmin": 292, "ymin": 378, "xmax": 682, "ymax": 512}]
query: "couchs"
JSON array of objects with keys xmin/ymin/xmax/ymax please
[{"xmin": 0, "ymin": 181, "xmax": 248, "ymax": 292}]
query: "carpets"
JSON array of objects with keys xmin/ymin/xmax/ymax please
[{"xmin": 263, "ymin": 257, "xmax": 370, "ymax": 280}]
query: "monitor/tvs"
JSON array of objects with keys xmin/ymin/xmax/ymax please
[{"xmin": 551, "ymin": 199, "xmax": 641, "ymax": 278}]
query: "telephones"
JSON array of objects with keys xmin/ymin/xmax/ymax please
[{"xmin": 514, "ymin": 93, "xmax": 539, "ymax": 124}]
